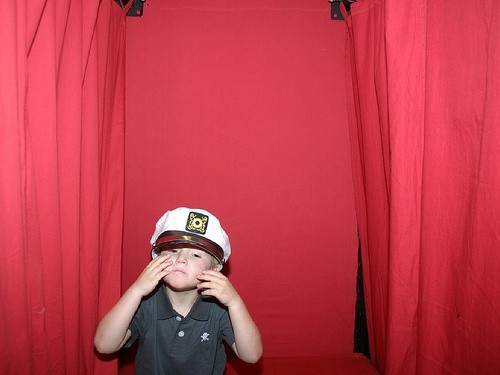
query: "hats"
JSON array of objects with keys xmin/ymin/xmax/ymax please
[{"xmin": 150, "ymin": 206, "xmax": 232, "ymax": 273}]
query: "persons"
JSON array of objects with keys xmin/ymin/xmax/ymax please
[{"xmin": 93, "ymin": 207, "xmax": 264, "ymax": 374}]
